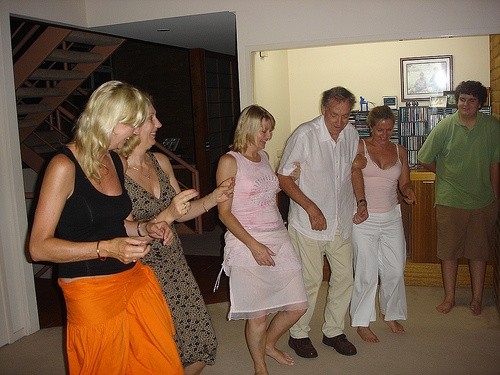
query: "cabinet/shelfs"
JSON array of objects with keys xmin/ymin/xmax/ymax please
[{"xmin": 397, "ymin": 169, "xmax": 470, "ymax": 265}]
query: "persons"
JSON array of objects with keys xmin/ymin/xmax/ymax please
[
  {"xmin": 214, "ymin": 104, "xmax": 308, "ymax": 375},
  {"xmin": 122, "ymin": 94, "xmax": 235, "ymax": 375},
  {"xmin": 418, "ymin": 80, "xmax": 500, "ymax": 316},
  {"xmin": 28, "ymin": 80, "xmax": 185, "ymax": 375},
  {"xmin": 277, "ymin": 86, "xmax": 367, "ymax": 359},
  {"xmin": 430, "ymin": 67, "xmax": 444, "ymax": 90},
  {"xmin": 410, "ymin": 72, "xmax": 426, "ymax": 94},
  {"xmin": 350, "ymin": 104, "xmax": 417, "ymax": 342}
]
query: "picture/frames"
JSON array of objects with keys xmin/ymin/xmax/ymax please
[{"xmin": 400, "ymin": 55, "xmax": 453, "ymax": 102}]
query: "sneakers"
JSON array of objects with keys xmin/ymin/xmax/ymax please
[
  {"xmin": 322, "ymin": 333, "xmax": 357, "ymax": 356},
  {"xmin": 288, "ymin": 334, "xmax": 318, "ymax": 358}
]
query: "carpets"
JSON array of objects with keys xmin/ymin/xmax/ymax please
[{"xmin": 0, "ymin": 281, "xmax": 499, "ymax": 374}]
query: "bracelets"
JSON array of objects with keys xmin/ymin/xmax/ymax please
[
  {"xmin": 96, "ymin": 240, "xmax": 106, "ymax": 261},
  {"xmin": 203, "ymin": 201, "xmax": 208, "ymax": 212},
  {"xmin": 357, "ymin": 199, "xmax": 367, "ymax": 206},
  {"xmin": 137, "ymin": 221, "xmax": 144, "ymax": 237}
]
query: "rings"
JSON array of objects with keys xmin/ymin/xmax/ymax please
[
  {"xmin": 133, "ymin": 260, "xmax": 136, "ymax": 262},
  {"xmin": 184, "ymin": 203, "xmax": 186, "ymax": 208},
  {"xmin": 186, "ymin": 209, "xmax": 188, "ymax": 212}
]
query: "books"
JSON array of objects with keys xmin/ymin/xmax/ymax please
[
  {"xmin": 429, "ymin": 107, "xmax": 457, "ymax": 133},
  {"xmin": 400, "ymin": 107, "xmax": 428, "ymax": 165},
  {"xmin": 387, "ymin": 110, "xmax": 399, "ymax": 144},
  {"xmin": 478, "ymin": 107, "xmax": 490, "ymax": 116},
  {"xmin": 347, "ymin": 111, "xmax": 371, "ymax": 139}
]
